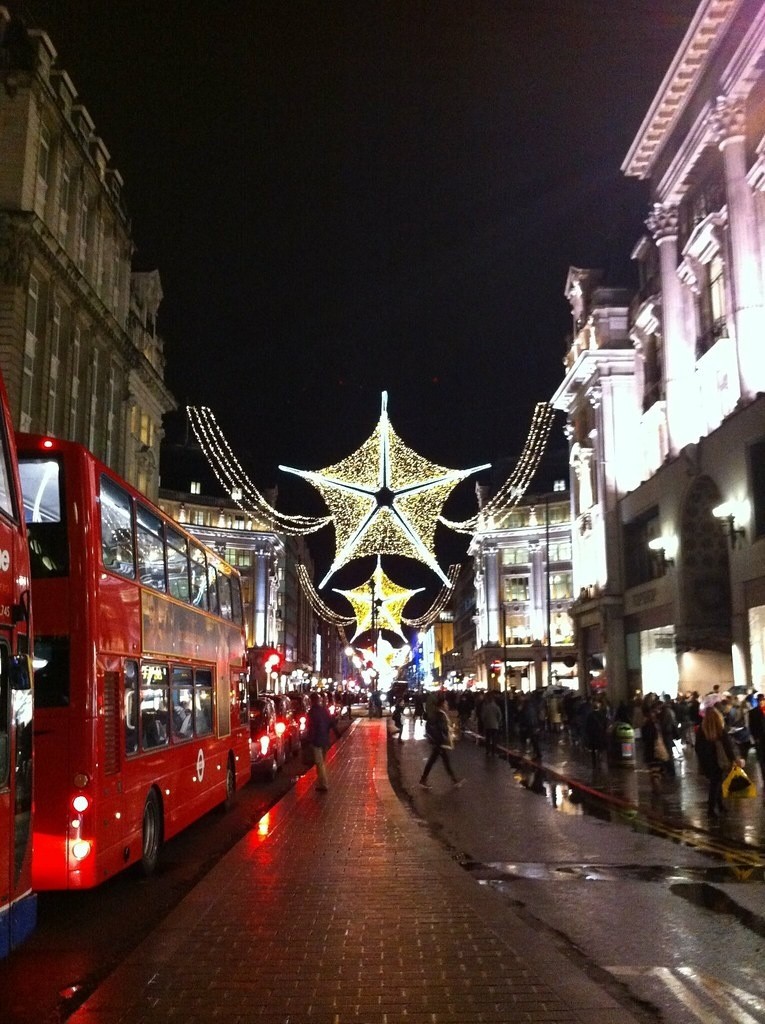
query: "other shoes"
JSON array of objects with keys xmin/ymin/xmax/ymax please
[
  {"xmin": 452, "ymin": 778, "xmax": 466, "ymax": 788},
  {"xmin": 419, "ymin": 780, "xmax": 433, "ymax": 789},
  {"xmin": 315, "ymin": 787, "xmax": 328, "ymax": 792}
]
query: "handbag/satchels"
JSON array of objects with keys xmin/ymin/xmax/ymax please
[{"xmin": 721, "ymin": 765, "xmax": 757, "ymax": 799}]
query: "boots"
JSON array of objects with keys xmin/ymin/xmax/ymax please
[
  {"xmin": 716, "ymin": 797, "xmax": 729, "ymax": 813},
  {"xmin": 707, "ymin": 800, "xmax": 719, "ymax": 820}
]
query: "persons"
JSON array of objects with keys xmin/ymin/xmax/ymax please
[
  {"xmin": 388, "ymin": 685, "xmax": 765, "ymax": 818},
  {"xmin": 335, "ymin": 690, "xmax": 367, "ymax": 720},
  {"xmin": 369, "ymin": 690, "xmax": 382, "ymax": 719},
  {"xmin": 104, "ymin": 528, "xmax": 142, "ymax": 577},
  {"xmin": 306, "ymin": 693, "xmax": 332, "ymax": 791},
  {"xmin": 126, "ymin": 700, "xmax": 209, "ymax": 751}
]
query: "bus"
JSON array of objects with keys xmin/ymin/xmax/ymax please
[
  {"xmin": 10, "ymin": 429, "xmax": 257, "ymax": 902},
  {"xmin": 0, "ymin": 367, "xmax": 41, "ymax": 966}
]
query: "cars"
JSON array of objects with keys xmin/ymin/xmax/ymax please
[{"xmin": 250, "ymin": 686, "xmax": 373, "ymax": 778}]
